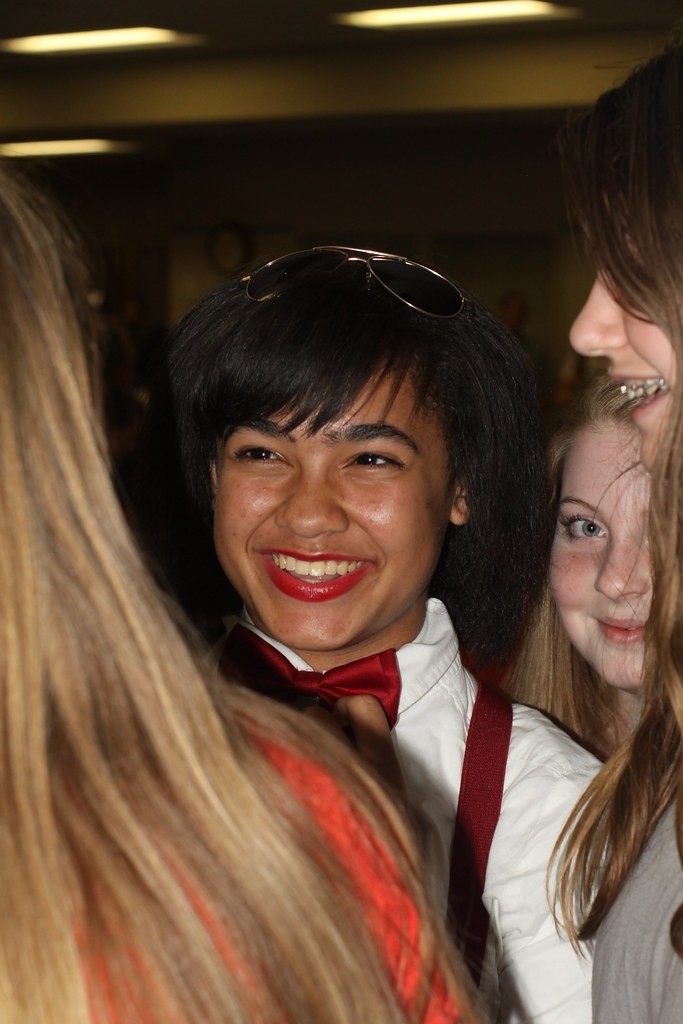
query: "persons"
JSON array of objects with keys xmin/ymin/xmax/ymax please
[
  {"xmin": 0, "ymin": 171, "xmax": 496, "ymax": 1024},
  {"xmin": 533, "ymin": 37, "xmax": 683, "ymax": 1024},
  {"xmin": 142, "ymin": 241, "xmax": 608, "ymax": 1024},
  {"xmin": 501, "ymin": 384, "xmax": 655, "ymax": 768}
]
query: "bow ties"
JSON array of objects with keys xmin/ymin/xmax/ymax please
[{"xmin": 219, "ymin": 623, "xmax": 401, "ymax": 732}]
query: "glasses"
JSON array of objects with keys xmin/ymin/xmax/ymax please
[{"xmin": 237, "ymin": 245, "xmax": 472, "ymax": 321}]
